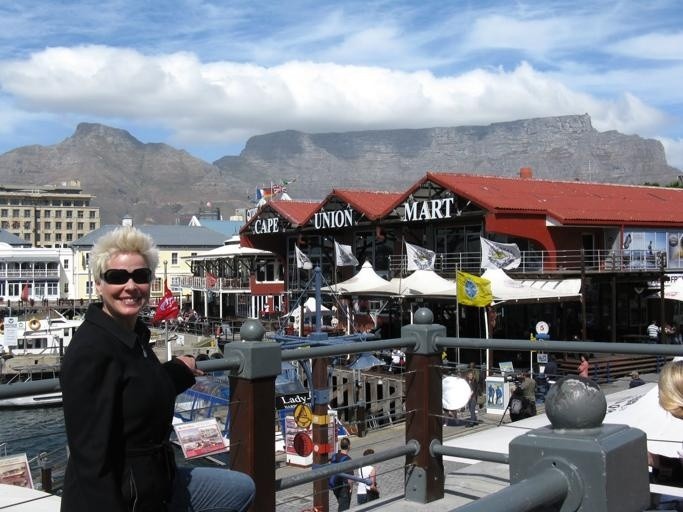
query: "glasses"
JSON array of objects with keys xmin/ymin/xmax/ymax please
[{"xmin": 100, "ymin": 268, "xmax": 151, "ymax": 284}]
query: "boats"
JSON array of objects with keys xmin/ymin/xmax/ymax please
[
  {"xmin": 0, "ymin": 307, "xmax": 164, "ymax": 408},
  {"xmin": 169, "ymin": 360, "xmax": 350, "ymax": 471}
]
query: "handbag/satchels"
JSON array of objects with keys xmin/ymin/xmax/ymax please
[{"xmin": 367, "ymin": 488, "xmax": 379, "ymax": 502}]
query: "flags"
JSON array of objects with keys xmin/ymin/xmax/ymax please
[
  {"xmin": 294, "ymin": 245, "xmax": 313, "ymax": 270},
  {"xmin": 481, "ymin": 236, "xmax": 522, "ymax": 271},
  {"xmin": 404, "ymin": 241, "xmax": 435, "ymax": 272},
  {"xmin": 333, "ymin": 241, "xmax": 360, "ymax": 267},
  {"xmin": 150, "ymin": 279, "xmax": 179, "ymax": 325},
  {"xmin": 246, "ymin": 176, "xmax": 298, "ymax": 204},
  {"xmin": 20, "ymin": 280, "xmax": 28, "ymax": 303},
  {"xmin": 456, "ymin": 269, "xmax": 492, "ymax": 308}
]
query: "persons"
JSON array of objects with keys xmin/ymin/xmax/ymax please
[
  {"xmin": 545, "ymin": 355, "xmax": 556, "ymax": 380},
  {"xmin": 3, "ymin": 289, "xmax": 230, "ymax": 336},
  {"xmin": 58, "ymin": 227, "xmax": 258, "ymax": 511},
  {"xmin": 647, "ymin": 320, "xmax": 683, "ymax": 345},
  {"xmin": 648, "ymin": 241, "xmax": 653, "ymax": 255},
  {"xmin": 365, "ymin": 315, "xmax": 382, "ymax": 334},
  {"xmin": 327, "ymin": 436, "xmax": 354, "ymax": 510},
  {"xmin": 644, "ymin": 360, "xmax": 682, "ymax": 490},
  {"xmin": 392, "ymin": 347, "xmax": 406, "ymax": 376},
  {"xmin": 578, "ymin": 353, "xmax": 589, "ymax": 377},
  {"xmin": 520, "ymin": 373, "xmax": 537, "ymax": 416},
  {"xmin": 671, "ymin": 235, "xmax": 682, "ymax": 261},
  {"xmin": 466, "ymin": 372, "xmax": 478, "ymax": 421},
  {"xmin": 355, "ymin": 448, "xmax": 377, "ymax": 504},
  {"xmin": 629, "ymin": 371, "xmax": 645, "ymax": 388}
]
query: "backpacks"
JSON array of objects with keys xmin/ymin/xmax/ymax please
[{"xmin": 329, "ymin": 454, "xmax": 348, "ymax": 488}]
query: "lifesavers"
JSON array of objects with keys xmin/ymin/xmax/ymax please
[{"xmin": 28, "ymin": 318, "xmax": 40, "ymax": 330}]
